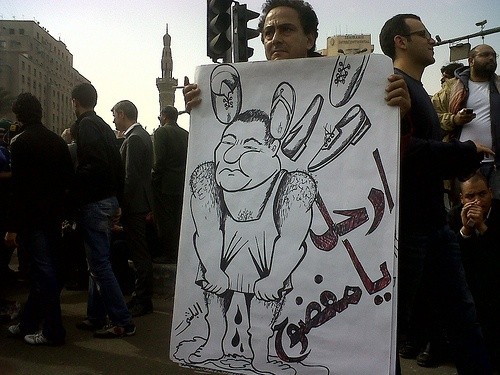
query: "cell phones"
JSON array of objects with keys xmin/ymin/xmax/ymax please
[{"xmin": 464, "ymin": 108, "xmax": 472, "ymax": 123}]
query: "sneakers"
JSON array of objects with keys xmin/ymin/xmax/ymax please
[
  {"xmin": 24, "ymin": 331, "xmax": 66, "ymax": 346},
  {"xmin": 93, "ymin": 321, "xmax": 137, "ymax": 338},
  {"xmin": 8, "ymin": 321, "xmax": 37, "ymax": 337},
  {"xmin": 75, "ymin": 319, "xmax": 109, "ymax": 330}
]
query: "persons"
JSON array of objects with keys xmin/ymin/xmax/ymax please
[
  {"xmin": 0, "ymin": 118, "xmax": 91, "ymax": 292},
  {"xmin": 71, "ymin": 84, "xmax": 138, "ymax": 337},
  {"xmin": 4, "ymin": 93, "xmax": 74, "ymax": 348},
  {"xmin": 152, "ymin": 107, "xmax": 188, "ymax": 263},
  {"xmin": 184, "ymin": 0, "xmax": 412, "ymax": 120},
  {"xmin": 108, "ymin": 98, "xmax": 156, "ymax": 318},
  {"xmin": 379, "ymin": 13, "xmax": 500, "ymax": 375}
]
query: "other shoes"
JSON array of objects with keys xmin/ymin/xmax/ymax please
[{"xmin": 125, "ymin": 299, "xmax": 153, "ymax": 318}]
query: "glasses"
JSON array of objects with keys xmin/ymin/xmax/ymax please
[
  {"xmin": 158, "ymin": 115, "xmax": 161, "ymax": 120},
  {"xmin": 406, "ymin": 29, "xmax": 431, "ymax": 40}
]
